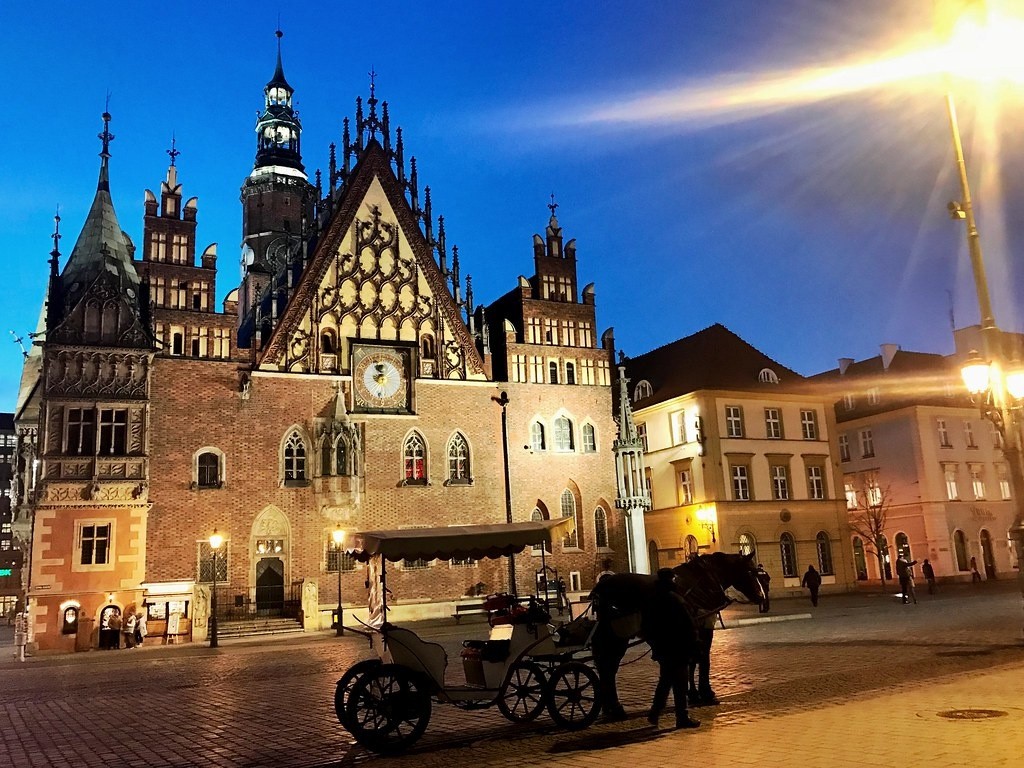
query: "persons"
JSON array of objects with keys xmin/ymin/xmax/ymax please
[
  {"xmin": 896, "ymin": 555, "xmax": 920, "ymax": 604},
  {"xmin": 108, "ymin": 609, "xmax": 143, "ymax": 650},
  {"xmin": 757, "ymin": 562, "xmax": 770, "ymax": 613},
  {"xmin": 801, "ymin": 565, "xmax": 822, "ymax": 607},
  {"xmin": 641, "ymin": 568, "xmax": 701, "ymax": 728},
  {"xmin": 922, "ymin": 559, "xmax": 938, "ymax": 594},
  {"xmin": 970, "ymin": 556, "xmax": 982, "ymax": 583}
]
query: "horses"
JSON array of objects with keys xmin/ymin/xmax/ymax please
[{"xmin": 586, "ymin": 547, "xmax": 773, "ymax": 727}]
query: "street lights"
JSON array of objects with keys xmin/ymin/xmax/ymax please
[
  {"xmin": 208, "ymin": 526, "xmax": 223, "ymax": 647},
  {"xmin": 331, "ymin": 527, "xmax": 345, "ymax": 636},
  {"xmin": 957, "ymin": 350, "xmax": 1023, "ymax": 584}
]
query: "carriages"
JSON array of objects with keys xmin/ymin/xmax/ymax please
[{"xmin": 334, "ymin": 518, "xmax": 772, "ymax": 755}]
